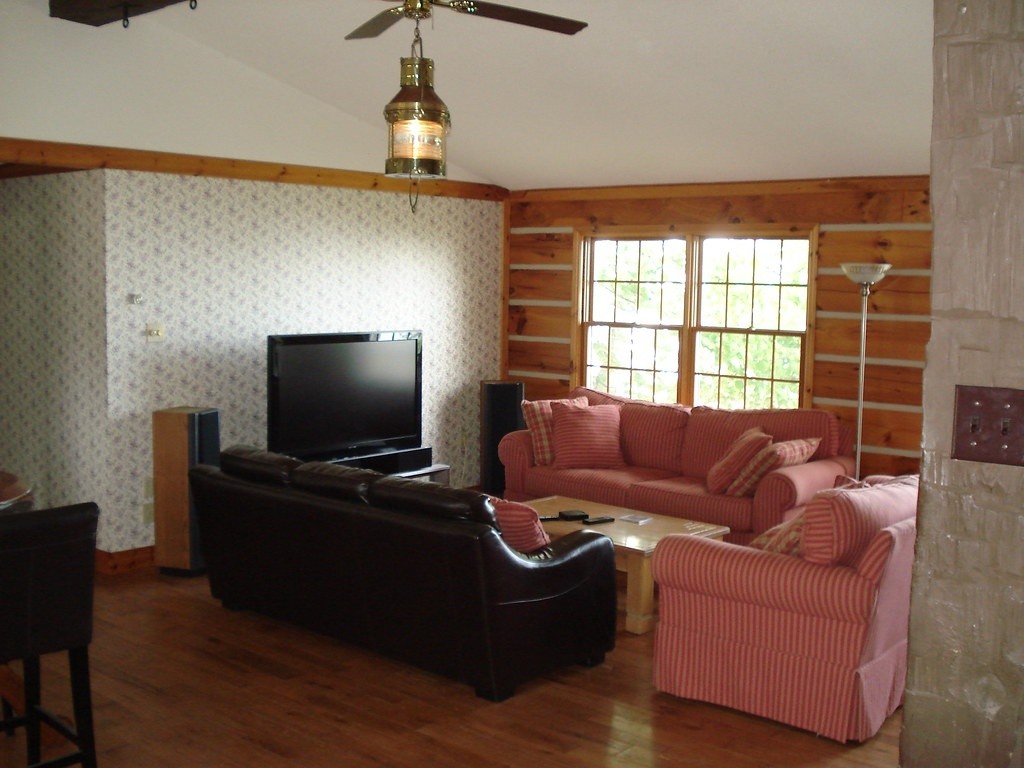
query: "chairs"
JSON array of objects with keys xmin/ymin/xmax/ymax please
[
  {"xmin": 654, "ymin": 472, "xmax": 934, "ymax": 744},
  {"xmin": 0, "ymin": 503, "xmax": 109, "ymax": 768}
]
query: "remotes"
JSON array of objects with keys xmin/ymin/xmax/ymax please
[{"xmin": 538, "ymin": 514, "xmax": 559, "ymax": 520}]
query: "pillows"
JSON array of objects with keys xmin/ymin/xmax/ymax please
[
  {"xmin": 482, "ymin": 493, "xmax": 550, "ymax": 555},
  {"xmin": 706, "ymin": 426, "xmax": 870, "ymax": 555},
  {"xmin": 522, "ymin": 395, "xmax": 591, "ymax": 466},
  {"xmin": 545, "ymin": 402, "xmax": 624, "ymax": 470}
]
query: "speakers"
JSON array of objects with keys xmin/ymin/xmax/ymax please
[
  {"xmin": 153, "ymin": 404, "xmax": 221, "ymax": 578},
  {"xmin": 480, "ymin": 379, "xmax": 526, "ymax": 492}
]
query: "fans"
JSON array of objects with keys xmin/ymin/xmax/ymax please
[{"xmin": 342, "ymin": 0, "xmax": 587, "ymax": 40}]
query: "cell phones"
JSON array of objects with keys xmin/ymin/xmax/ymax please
[{"xmin": 583, "ymin": 516, "xmax": 614, "ymax": 525}]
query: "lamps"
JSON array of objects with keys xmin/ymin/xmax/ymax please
[
  {"xmin": 833, "ymin": 261, "xmax": 891, "ymax": 480},
  {"xmin": 382, "ymin": 26, "xmax": 451, "ymax": 213}
]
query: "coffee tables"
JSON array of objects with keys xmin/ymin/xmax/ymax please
[{"xmin": 513, "ymin": 493, "xmax": 727, "ymax": 635}]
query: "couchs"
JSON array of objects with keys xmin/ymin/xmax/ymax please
[
  {"xmin": 497, "ymin": 385, "xmax": 860, "ymax": 543},
  {"xmin": 193, "ymin": 443, "xmax": 617, "ymax": 704}
]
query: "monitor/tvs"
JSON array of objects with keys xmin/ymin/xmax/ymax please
[{"xmin": 267, "ymin": 329, "xmax": 422, "ymax": 462}]
